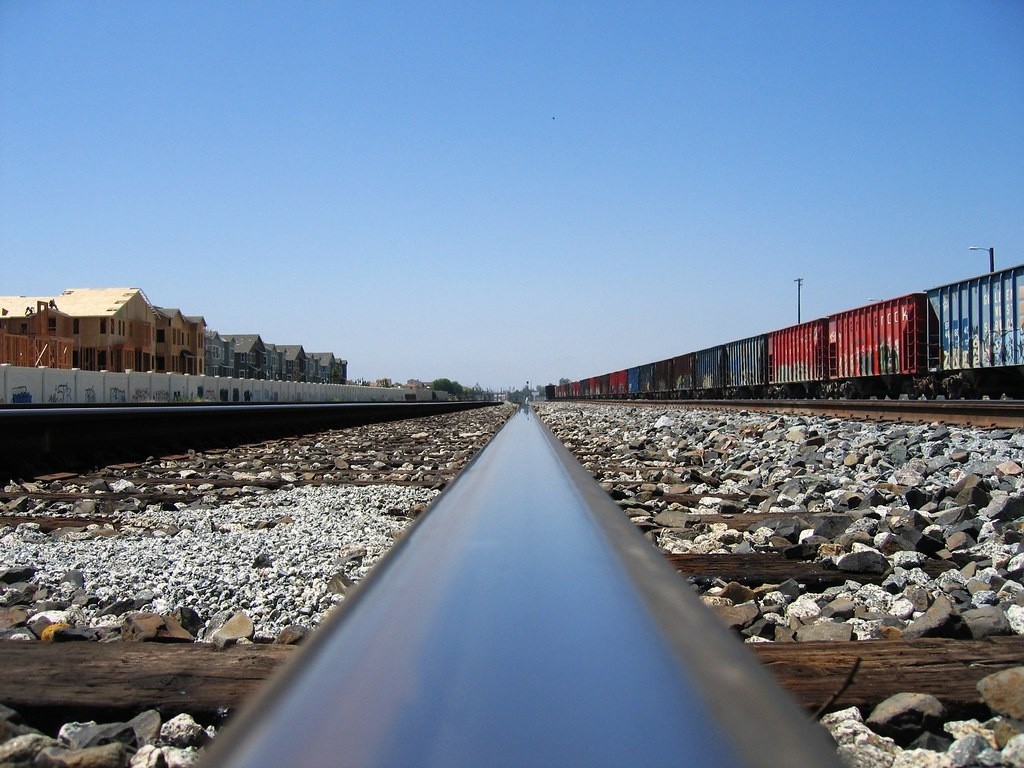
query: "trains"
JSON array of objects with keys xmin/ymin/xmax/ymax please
[{"xmin": 554, "ymin": 265, "xmax": 1024, "ymax": 401}]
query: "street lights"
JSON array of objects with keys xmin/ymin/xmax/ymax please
[
  {"xmin": 794, "ymin": 278, "xmax": 804, "ymax": 325},
  {"xmin": 968, "ymin": 247, "xmax": 994, "ymax": 273}
]
